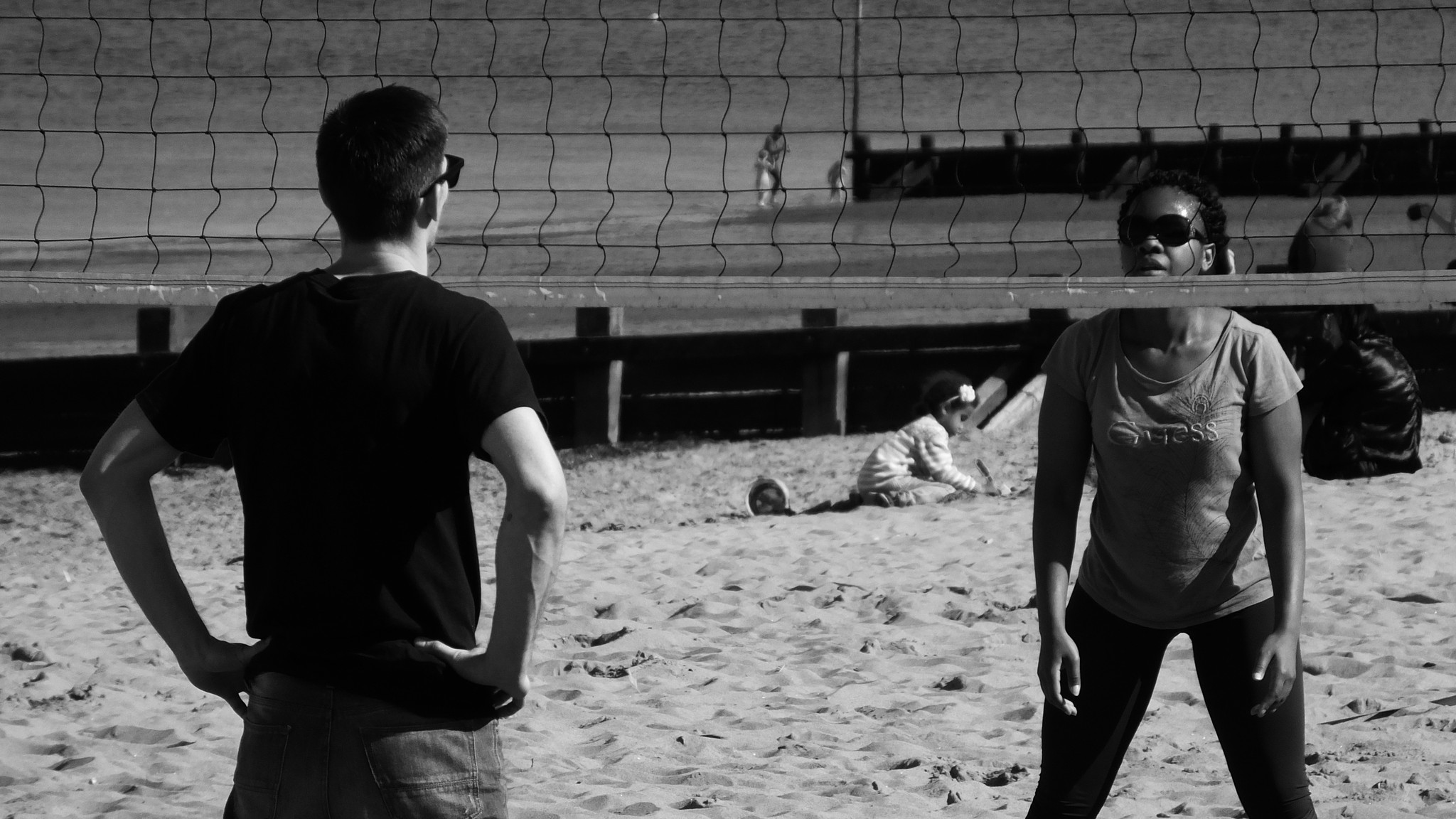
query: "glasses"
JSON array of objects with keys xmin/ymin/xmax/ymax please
[
  {"xmin": 1121, "ymin": 213, "xmax": 1207, "ymax": 248},
  {"xmin": 419, "ymin": 154, "xmax": 464, "ymax": 198}
]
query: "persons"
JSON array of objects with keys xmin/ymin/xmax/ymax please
[
  {"xmin": 848, "ymin": 370, "xmax": 988, "ymax": 507},
  {"xmin": 1022, "ymin": 168, "xmax": 1319, "ymax": 819},
  {"xmin": 79, "ymin": 83, "xmax": 569, "ymax": 819},
  {"xmin": 754, "ymin": 124, "xmax": 792, "ymax": 206},
  {"xmin": 1289, "ymin": 199, "xmax": 1423, "ymax": 480},
  {"xmin": 826, "ymin": 161, "xmax": 846, "ymax": 203}
]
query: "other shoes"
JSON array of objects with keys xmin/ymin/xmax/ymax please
[{"xmin": 869, "ymin": 492, "xmax": 890, "ymax": 507}]
